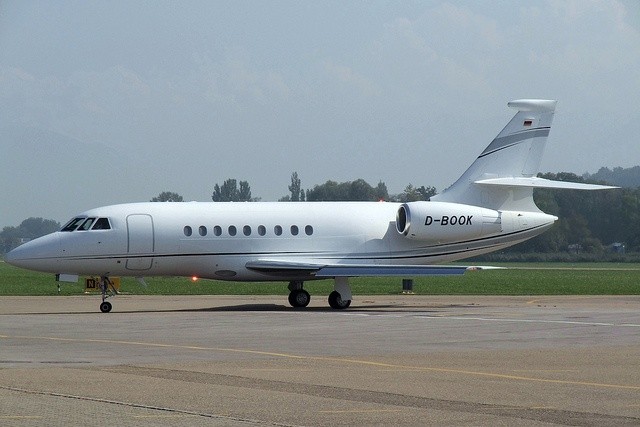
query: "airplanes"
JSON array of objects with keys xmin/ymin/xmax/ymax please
[{"xmin": 5, "ymin": 100, "xmax": 622, "ymax": 313}]
